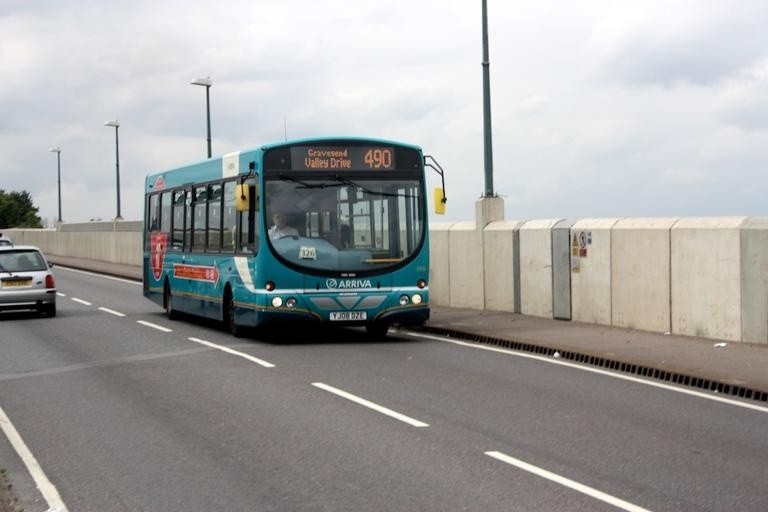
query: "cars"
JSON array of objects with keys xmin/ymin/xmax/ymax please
[
  {"xmin": 0, "ymin": 246, "xmax": 59, "ymax": 322},
  {"xmin": 0, "ymin": 235, "xmax": 16, "ymax": 246}
]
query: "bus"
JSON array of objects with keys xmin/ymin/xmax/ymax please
[{"xmin": 143, "ymin": 137, "xmax": 448, "ymax": 345}]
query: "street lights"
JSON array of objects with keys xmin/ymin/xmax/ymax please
[
  {"xmin": 47, "ymin": 146, "xmax": 66, "ymax": 222},
  {"xmin": 190, "ymin": 77, "xmax": 214, "ymax": 160},
  {"xmin": 103, "ymin": 121, "xmax": 126, "ymax": 219}
]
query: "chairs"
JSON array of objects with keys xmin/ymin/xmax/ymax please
[{"xmin": 320, "ymin": 232, "xmax": 343, "ymax": 250}]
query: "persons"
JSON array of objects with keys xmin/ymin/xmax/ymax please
[{"xmin": 268, "ymin": 212, "xmax": 300, "ymax": 241}]
